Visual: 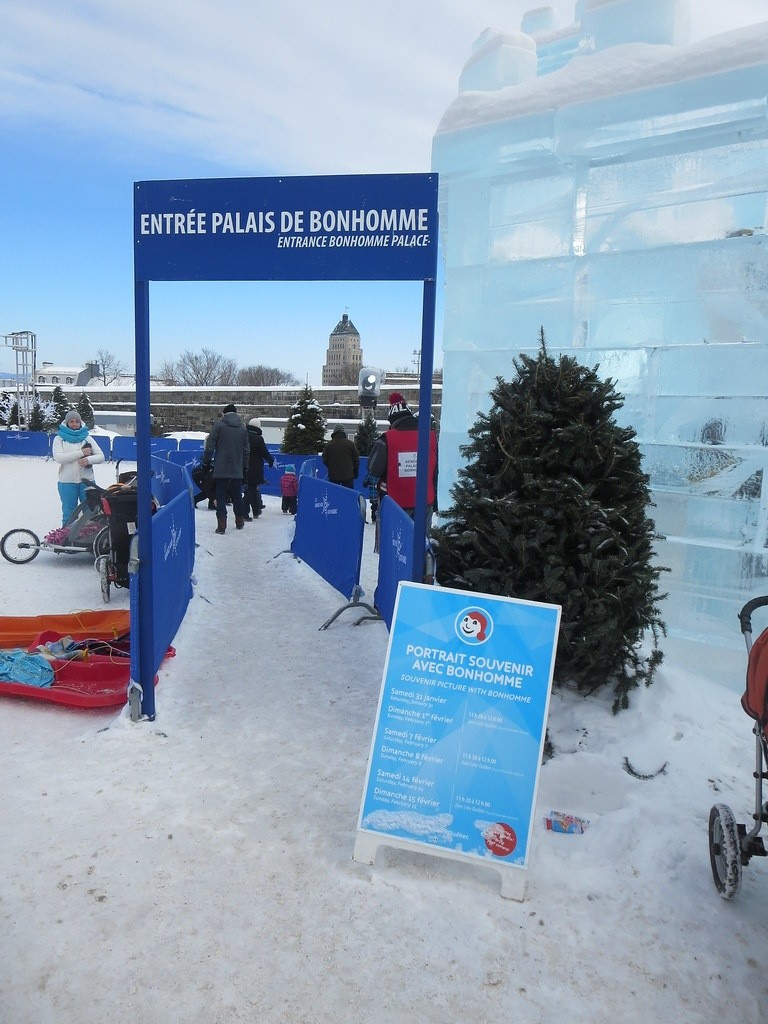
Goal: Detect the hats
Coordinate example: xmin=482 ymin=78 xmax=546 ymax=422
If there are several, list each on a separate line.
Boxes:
xmin=222 ymin=403 xmax=237 ymax=413
xmin=333 ymin=424 xmax=344 ymax=433
xmin=65 ymin=411 xmax=81 ymax=427
xmin=284 ymin=464 xmax=296 ymax=475
xmin=387 ymin=393 xmax=414 ymax=424
xmin=249 ymin=418 xmax=262 ymax=427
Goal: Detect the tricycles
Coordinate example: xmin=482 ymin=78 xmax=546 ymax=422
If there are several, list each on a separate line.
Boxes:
xmin=0 ymin=455 xmax=138 ymax=603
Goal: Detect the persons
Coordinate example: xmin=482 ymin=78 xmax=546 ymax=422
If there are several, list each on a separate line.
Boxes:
xmin=323 ymin=423 xmax=360 ymax=490
xmin=192 ymin=404 xmax=273 ymax=534
xmin=368 ymin=394 xmax=438 ymax=586
xmin=281 ymin=465 xmax=298 ymax=515
xmin=53 ymin=411 xmax=105 ymax=554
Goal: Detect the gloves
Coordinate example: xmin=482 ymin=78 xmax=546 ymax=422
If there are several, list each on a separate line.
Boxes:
xmin=268 ymin=461 xmax=275 ymax=468
xmin=353 ymin=468 xmax=359 ymax=479
xmin=243 ymin=470 xmax=249 ymax=480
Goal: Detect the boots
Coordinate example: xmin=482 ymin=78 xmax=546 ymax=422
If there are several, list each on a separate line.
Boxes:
xmin=215 ymin=518 xmax=226 ymax=533
xmin=236 ymin=515 xmax=244 ymax=529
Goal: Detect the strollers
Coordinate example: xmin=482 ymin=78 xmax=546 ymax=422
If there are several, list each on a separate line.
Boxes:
xmin=709 ymin=595 xmax=768 ymax=899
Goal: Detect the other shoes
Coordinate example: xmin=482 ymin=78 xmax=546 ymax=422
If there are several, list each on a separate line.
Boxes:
xmin=195 ymin=502 xmax=198 ymax=508
xmin=283 ymin=509 xmax=287 ymax=513
xmin=261 ymin=504 xmax=266 ymax=510
xmin=253 ymin=509 xmax=262 ymax=518
xmin=208 ymin=502 xmax=217 ymax=509
xmin=243 ymin=517 xmax=252 ymax=522
